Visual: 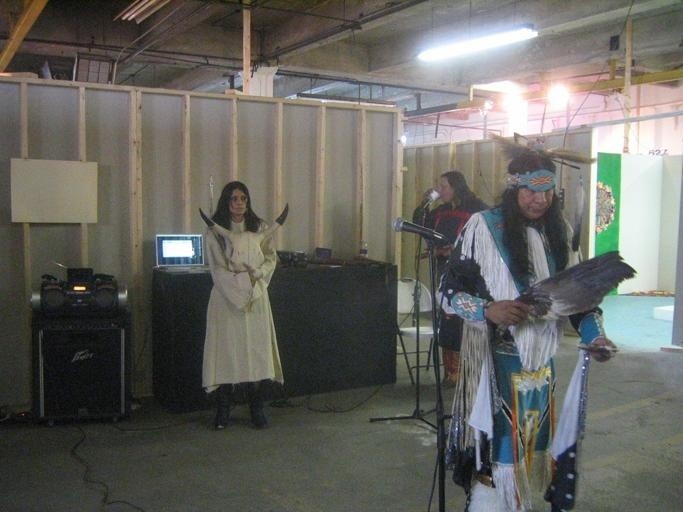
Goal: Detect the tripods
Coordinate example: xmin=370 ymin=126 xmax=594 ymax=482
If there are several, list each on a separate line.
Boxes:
xmin=368 ymin=242 xmax=440 ymax=431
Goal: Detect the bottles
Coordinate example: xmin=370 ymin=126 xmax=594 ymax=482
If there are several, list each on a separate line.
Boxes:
xmin=358 ymin=239 xmax=367 ymax=263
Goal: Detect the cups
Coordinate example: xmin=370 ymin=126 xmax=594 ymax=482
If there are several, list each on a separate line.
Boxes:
xmin=429 ymin=191 xmax=439 ymax=201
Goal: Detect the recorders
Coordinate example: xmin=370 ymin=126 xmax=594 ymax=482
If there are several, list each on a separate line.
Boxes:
xmin=31 ymin=267 xmax=128 ymax=318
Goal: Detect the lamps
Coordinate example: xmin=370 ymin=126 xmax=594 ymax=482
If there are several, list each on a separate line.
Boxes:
xmin=415 ymin=1 xmax=540 ymax=67
xmin=113 ymin=0 xmax=171 ymax=25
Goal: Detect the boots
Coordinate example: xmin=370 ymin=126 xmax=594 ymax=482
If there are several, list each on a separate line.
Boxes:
xmin=249 ymin=384 xmax=268 ymax=430
xmin=212 ymin=380 xmax=231 ymax=430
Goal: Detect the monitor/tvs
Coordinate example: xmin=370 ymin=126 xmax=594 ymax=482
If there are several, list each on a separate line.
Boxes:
xmin=155 ymin=232 xmax=204 ymax=273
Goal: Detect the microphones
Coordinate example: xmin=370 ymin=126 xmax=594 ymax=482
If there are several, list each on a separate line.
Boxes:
xmin=391 ymin=216 xmax=445 ymax=242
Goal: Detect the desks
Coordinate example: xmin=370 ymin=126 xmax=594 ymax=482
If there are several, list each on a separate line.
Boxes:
xmin=145 ymin=250 xmax=398 ymax=417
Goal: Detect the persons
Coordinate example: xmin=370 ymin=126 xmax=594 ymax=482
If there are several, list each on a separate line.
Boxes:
xmin=434 ymin=147 xmax=622 ymax=511
xmin=411 ymin=171 xmax=495 ymax=389
xmin=203 ymin=181 xmax=282 ymax=430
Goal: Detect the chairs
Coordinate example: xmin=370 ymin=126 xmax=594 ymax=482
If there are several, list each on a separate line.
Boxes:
xmin=393 ymin=274 xmax=445 ymax=392
